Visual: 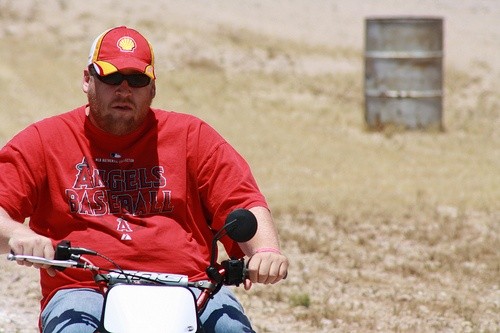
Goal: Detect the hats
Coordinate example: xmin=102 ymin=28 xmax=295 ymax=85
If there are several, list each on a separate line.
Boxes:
xmin=87 ymin=25 xmax=157 ymax=80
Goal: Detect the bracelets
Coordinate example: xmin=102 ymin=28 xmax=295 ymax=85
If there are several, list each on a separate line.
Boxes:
xmin=251 ymin=248 xmax=282 ymax=255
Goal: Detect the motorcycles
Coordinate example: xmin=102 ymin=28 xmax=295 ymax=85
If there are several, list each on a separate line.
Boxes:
xmin=7 ymin=208 xmax=287 ymax=333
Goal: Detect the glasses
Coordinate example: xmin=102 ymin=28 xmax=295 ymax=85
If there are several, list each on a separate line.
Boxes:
xmin=95 ymin=72 xmax=151 ymax=88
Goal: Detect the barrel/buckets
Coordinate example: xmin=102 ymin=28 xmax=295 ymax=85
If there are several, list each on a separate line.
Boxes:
xmin=365 ymin=18 xmax=444 ymax=130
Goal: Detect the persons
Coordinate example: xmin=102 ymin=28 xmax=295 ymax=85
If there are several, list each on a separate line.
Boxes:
xmin=0 ymin=26 xmax=288 ymax=333
